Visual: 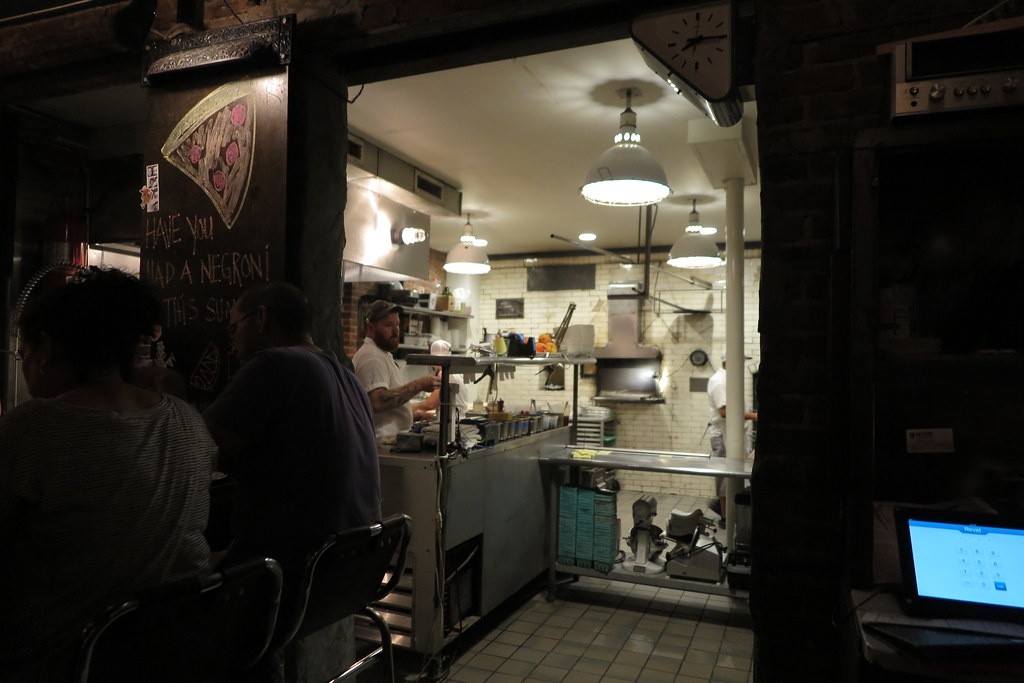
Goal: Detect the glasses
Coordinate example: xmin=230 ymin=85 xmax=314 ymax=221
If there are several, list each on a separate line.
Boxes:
xmin=226 ymin=310 xmax=257 ymax=335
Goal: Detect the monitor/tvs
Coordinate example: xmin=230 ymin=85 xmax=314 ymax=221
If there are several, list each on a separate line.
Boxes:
xmin=892 ymin=505 xmax=1024 ymax=625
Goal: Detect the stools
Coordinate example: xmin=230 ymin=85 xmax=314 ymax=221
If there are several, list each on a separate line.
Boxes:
xmin=264 ymin=512 xmax=413 ymax=683
xmin=70 ymin=556 xmax=284 ymax=683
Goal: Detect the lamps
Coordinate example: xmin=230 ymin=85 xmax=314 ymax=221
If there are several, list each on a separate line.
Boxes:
xmin=442 ymin=212 xmax=491 ymax=275
xmin=665 ymin=199 xmax=725 ymax=270
xmin=577 ymin=87 xmax=673 ymax=207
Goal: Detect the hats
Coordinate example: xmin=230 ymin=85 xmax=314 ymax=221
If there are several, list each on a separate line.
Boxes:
xmin=721 ymin=349 xmax=752 ymax=362
xmin=363 ymin=300 xmax=403 ymax=320
xmin=431 ymin=339 xmax=452 ymax=356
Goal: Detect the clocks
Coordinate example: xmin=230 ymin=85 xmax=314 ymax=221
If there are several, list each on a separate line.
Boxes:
xmin=628 ymin=0 xmax=744 ymax=128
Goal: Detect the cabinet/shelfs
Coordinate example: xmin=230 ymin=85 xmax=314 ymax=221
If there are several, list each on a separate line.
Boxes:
xmin=356 ymin=302 xmax=475 ymax=360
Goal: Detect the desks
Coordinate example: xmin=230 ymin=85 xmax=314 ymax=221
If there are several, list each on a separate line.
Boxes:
xmin=538 ymin=447 xmax=753 ymax=603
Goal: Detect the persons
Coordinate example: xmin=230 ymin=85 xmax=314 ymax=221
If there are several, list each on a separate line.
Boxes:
xmin=411 ymin=341 xmax=469 ymax=421
xmin=0 ymin=267 xmax=381 ymax=683
xmin=706 ymin=351 xmax=758 ymax=529
xmin=354 ymin=300 xmax=442 ymax=439
